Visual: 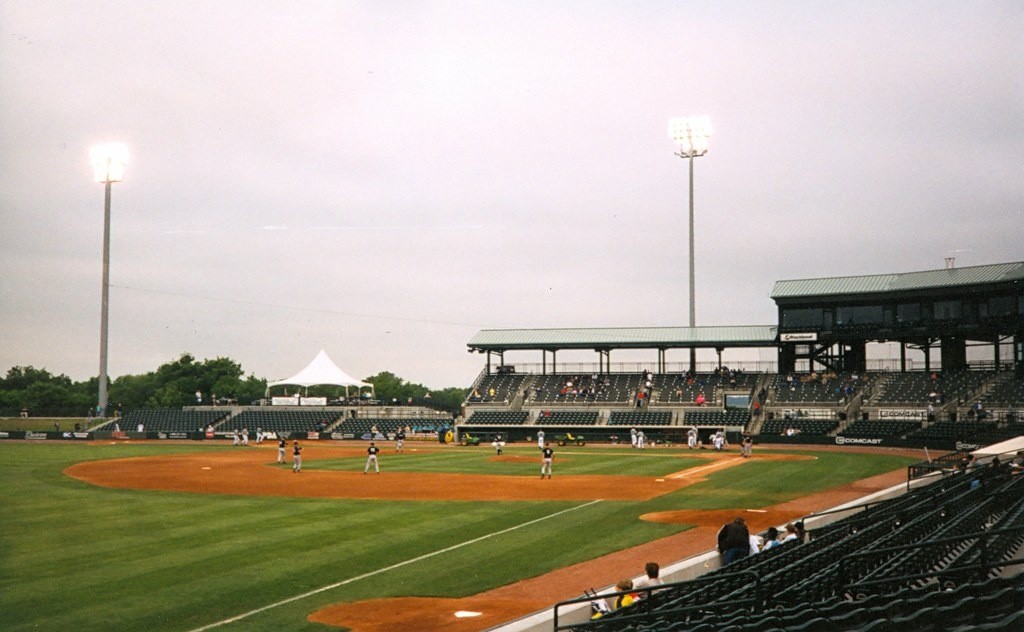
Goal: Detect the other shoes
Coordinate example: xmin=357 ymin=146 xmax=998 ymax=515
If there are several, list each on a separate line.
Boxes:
xmin=540 ymin=474 xmax=544 ymax=479
xmin=256 ymin=442 xmax=263 ymax=445
xmin=277 ymin=462 xmax=286 ymax=464
xmin=396 ymin=450 xmax=403 ymax=453
xmin=377 ymin=472 xmax=379 ymax=475
xmin=637 ymin=448 xmax=646 ymax=450
xmin=689 ymin=447 xmax=693 ymax=449
xmin=548 ymin=475 xmax=552 ymax=479
xmin=539 ymin=447 xmax=542 ymax=451
xmin=363 ymin=471 xmax=366 ymax=474
xmin=293 ymin=470 xmax=303 ymax=473
xmin=632 ymin=445 xmax=635 ymax=447
xmin=233 ymin=442 xmax=247 ymax=446
xmin=497 ymin=449 xmax=504 ymax=455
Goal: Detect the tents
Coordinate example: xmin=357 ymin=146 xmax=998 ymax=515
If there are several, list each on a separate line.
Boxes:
xmin=266 ymin=350 xmax=374 ymax=406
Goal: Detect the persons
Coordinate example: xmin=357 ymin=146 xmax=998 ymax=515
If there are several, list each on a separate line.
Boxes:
xmin=365 ymin=442 xmax=379 ymax=473
xmin=371 ymin=425 xmax=410 ymax=453
xmin=256 ymin=424 xmax=263 ymax=444
xmin=614 ymin=563 xmax=664 ymax=611
xmin=523 ymin=386 xmax=545 ymax=404
xmin=293 ymin=442 xmax=302 ymax=472
xmin=493 ymin=438 xmax=502 ymax=454
xmin=233 ymin=425 xmax=249 ymax=446
xmin=212 ymin=393 xmax=232 ymax=406
xmin=561 ymin=372 xmax=610 ymax=398
xmin=537 ymin=429 xmax=553 ymax=479
xmin=278 ymin=437 xmax=287 ymax=463
xmin=754 ymin=370 xmax=867 ymax=437
xmin=928 ymin=402 xmax=934 ymax=413
xmin=362 ymin=390 xmax=370 ymax=401
xmin=630 ymin=427 xmax=644 ymax=448
xmin=974 ymin=400 xmax=983 ymax=411
xmin=638 ymin=365 xmax=740 ymax=408
xmin=20 ymin=403 xmax=144 ymax=432
xmin=688 ymin=424 xmax=753 ymax=459
xmin=716 ymin=518 xmax=804 ymax=564
xmin=196 ymin=390 xmax=202 ymax=403
xmin=207 ymin=425 xmax=214 ymax=432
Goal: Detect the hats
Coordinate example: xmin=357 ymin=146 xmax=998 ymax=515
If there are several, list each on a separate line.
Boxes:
xmin=785 ymin=523 xmax=793 ymax=531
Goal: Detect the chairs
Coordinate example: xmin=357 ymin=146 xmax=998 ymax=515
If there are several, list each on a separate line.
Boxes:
xmin=574 ymin=469 xmax=1024 ymax=632
xmin=465 ymin=368 xmax=1024 ymax=448
xmin=95 ymin=406 xmax=455 ymax=433
